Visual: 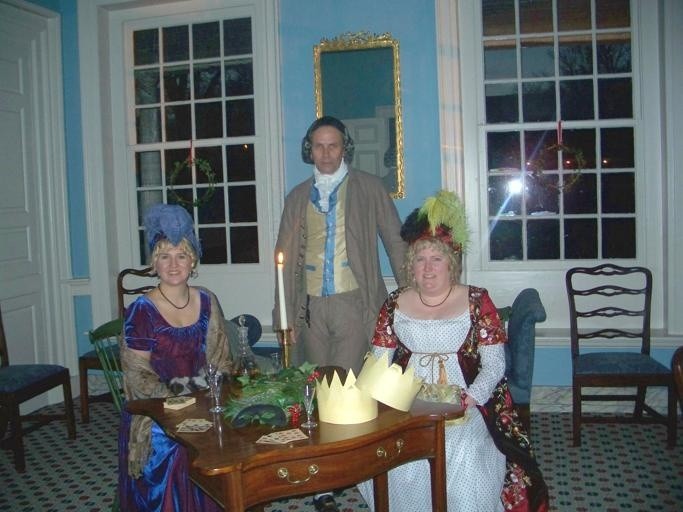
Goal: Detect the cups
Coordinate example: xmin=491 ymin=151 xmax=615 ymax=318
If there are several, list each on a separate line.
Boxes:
xmin=271 ymin=352 xmax=282 ymax=368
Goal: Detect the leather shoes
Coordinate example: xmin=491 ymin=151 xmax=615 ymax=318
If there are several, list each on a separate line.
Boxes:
xmin=312 ymin=493 xmax=340 ymax=512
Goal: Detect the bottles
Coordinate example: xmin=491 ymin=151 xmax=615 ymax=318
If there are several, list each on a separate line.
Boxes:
xmin=226 ymin=316 xmax=261 ymax=404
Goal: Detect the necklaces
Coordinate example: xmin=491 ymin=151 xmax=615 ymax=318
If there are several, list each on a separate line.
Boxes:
xmin=157 ymin=283 xmax=191 ymax=309
xmin=416 ymin=288 xmax=452 ymax=306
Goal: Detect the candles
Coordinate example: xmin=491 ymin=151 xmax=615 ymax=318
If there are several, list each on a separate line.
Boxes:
xmin=276 ymin=250 xmax=288 ymax=331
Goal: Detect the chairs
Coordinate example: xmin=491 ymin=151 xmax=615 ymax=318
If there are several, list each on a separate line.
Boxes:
xmin=565 ymin=261 xmax=679 ymax=450
xmin=87 ymin=316 xmax=129 ymax=511
xmin=211 ymin=292 xmax=283 ymax=379
xmin=497 ymin=287 xmax=547 ymax=445
xmin=78 ymin=263 xmax=160 ymax=426
xmin=0 ymin=308 xmax=76 ymax=474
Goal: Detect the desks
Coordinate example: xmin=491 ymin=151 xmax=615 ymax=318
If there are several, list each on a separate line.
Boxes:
xmin=123 ymin=364 xmax=465 ymax=512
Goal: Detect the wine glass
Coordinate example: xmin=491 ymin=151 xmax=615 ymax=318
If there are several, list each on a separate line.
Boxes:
xmin=201 ymin=361 xmax=225 ymax=413
xmin=301 ymin=385 xmax=317 ymax=429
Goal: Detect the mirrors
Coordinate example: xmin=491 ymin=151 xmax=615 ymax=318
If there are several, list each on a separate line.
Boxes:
xmin=310 ymin=27 xmax=407 ymax=202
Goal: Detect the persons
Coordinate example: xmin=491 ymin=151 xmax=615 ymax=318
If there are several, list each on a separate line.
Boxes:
xmin=273 ymin=115 xmax=409 ymax=511
xmin=113 ymin=203 xmax=272 ymax=512
xmin=355 ymin=188 xmax=549 ymax=512
